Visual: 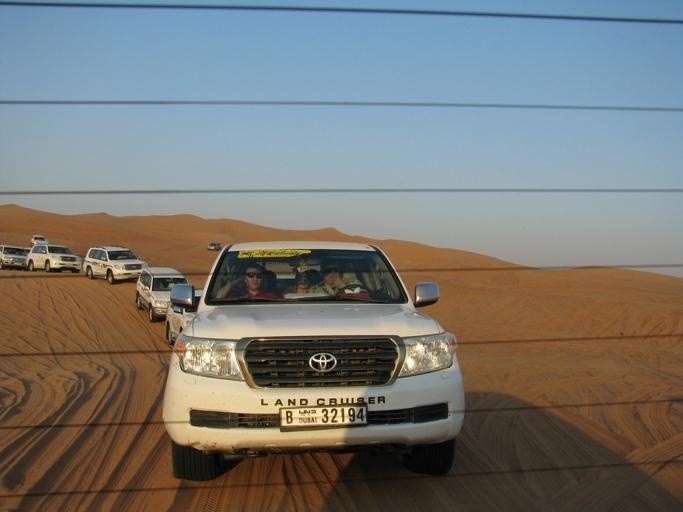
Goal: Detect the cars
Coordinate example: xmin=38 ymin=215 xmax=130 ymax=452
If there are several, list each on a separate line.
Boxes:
xmin=29 ymin=235 xmax=47 ymax=245
xmin=207 ymin=242 xmax=220 ymax=251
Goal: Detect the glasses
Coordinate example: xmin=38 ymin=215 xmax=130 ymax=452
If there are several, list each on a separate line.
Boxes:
xmin=246 ymin=272 xmax=263 ymax=278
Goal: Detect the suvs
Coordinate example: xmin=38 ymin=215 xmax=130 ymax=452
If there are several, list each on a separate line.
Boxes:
xmin=0 ymin=245 xmax=30 ymax=269
xmin=135 ymin=266 xmax=188 ymax=323
xmin=162 ymin=289 xmax=204 ymax=345
xmin=24 ymin=244 xmax=81 ymax=273
xmin=160 ymin=241 xmax=466 ymax=480
xmin=82 ymin=246 xmax=147 ymax=285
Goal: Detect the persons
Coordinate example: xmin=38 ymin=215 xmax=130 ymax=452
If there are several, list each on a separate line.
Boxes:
xmin=215 ymin=263 xmax=277 ymax=301
xmin=278 ymin=262 xmax=321 ymax=300
xmin=309 ymin=257 xmax=365 ymax=298
